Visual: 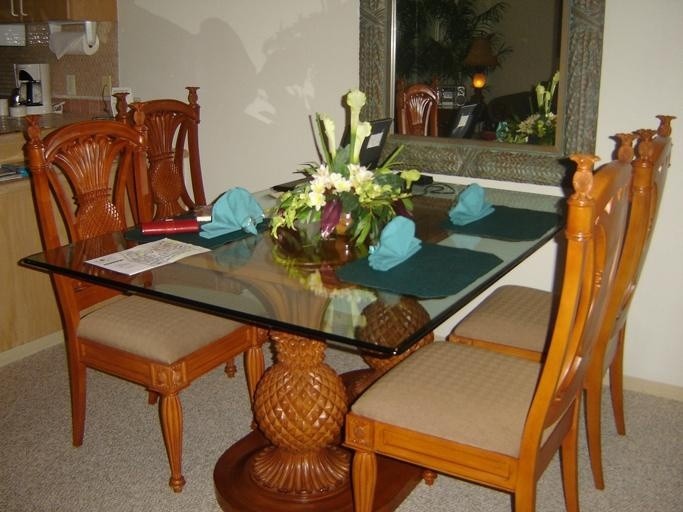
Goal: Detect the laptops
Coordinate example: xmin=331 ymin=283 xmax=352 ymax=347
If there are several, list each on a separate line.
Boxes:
xmin=451 ymin=104 xmax=477 ymax=136
xmin=273 ymin=118 xmax=392 ymax=192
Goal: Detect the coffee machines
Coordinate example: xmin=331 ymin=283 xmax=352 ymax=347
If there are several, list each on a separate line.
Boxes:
xmin=14 ymin=63 xmax=52 ymax=115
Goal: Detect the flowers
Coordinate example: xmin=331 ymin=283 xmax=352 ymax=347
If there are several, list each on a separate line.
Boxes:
xmin=516 ymin=70 xmax=559 ymax=139
xmin=271 ymin=90 xmax=419 ymax=253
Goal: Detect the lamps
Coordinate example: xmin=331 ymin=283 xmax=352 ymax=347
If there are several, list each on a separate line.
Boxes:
xmin=460 ymin=37 xmax=499 ymax=123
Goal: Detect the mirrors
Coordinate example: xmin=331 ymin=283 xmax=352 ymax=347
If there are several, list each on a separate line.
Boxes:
xmin=357 ymin=0 xmax=606 ymax=188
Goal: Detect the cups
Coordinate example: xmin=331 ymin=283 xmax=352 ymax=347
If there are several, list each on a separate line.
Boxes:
xmin=1 ymin=99 xmax=9 ymax=116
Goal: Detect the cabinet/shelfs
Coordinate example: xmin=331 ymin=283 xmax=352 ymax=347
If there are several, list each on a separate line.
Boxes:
xmin=0 ymin=142 xmax=190 ymax=368
xmin=0 ymin=0 xmax=116 ymax=26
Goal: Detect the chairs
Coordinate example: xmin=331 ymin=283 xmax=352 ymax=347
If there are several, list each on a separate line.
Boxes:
xmin=343 ymin=131 xmax=639 ymax=512
xmin=394 ymin=75 xmax=440 ymax=139
xmin=109 ymin=86 xmax=236 ymax=377
xmin=23 ymin=101 xmax=269 ymax=492
xmin=445 ymin=114 xmax=677 ymax=491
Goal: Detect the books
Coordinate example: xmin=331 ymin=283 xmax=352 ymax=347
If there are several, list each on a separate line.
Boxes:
xmin=195 ymin=203 xmax=211 ymax=222
xmin=0 ymin=163 xmax=29 ymax=182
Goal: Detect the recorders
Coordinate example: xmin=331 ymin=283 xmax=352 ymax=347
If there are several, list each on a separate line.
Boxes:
xmin=436 ymin=85 xmax=465 ymax=107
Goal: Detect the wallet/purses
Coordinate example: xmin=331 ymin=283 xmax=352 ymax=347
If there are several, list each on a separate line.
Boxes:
xmin=142 ymin=220 xmax=200 ymax=236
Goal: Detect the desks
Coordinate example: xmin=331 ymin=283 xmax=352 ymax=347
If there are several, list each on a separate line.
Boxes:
xmin=17 ymin=168 xmax=569 ymax=511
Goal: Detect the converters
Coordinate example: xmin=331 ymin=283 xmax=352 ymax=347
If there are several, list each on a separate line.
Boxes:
xmin=397 ymin=172 xmax=433 ymax=185
